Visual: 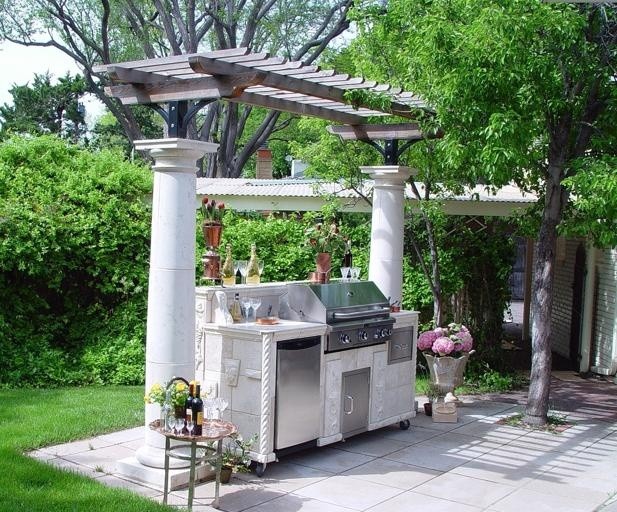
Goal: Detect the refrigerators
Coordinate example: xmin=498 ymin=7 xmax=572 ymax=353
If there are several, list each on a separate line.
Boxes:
xmin=274 ymin=335 xmax=322 ymax=450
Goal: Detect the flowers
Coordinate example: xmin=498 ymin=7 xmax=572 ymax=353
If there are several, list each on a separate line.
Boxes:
xmin=201 ymin=197 xmax=224 ymax=225
xmin=145 ymin=380 xmax=189 ymax=416
xmin=417 ymin=322 xmax=474 ymax=358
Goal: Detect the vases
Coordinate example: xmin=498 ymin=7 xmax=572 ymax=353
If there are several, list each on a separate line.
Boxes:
xmin=202 ymin=223 xmax=221 ymax=247
xmin=158 ymin=405 xmax=173 ymax=427
xmin=425 ymin=351 xmax=472 ymax=402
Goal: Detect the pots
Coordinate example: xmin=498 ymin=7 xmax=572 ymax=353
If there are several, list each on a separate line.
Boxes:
xmin=257 ymin=304 xmax=280 ymax=325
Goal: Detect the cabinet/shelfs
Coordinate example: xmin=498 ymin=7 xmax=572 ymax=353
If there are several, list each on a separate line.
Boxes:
xmin=369 ymin=352 xmax=417 ymax=432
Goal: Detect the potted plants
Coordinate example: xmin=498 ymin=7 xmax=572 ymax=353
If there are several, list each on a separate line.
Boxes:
xmin=301 ymin=224 xmax=347 ymax=282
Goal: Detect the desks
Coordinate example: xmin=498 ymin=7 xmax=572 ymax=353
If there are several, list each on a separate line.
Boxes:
xmin=150 ymin=419 xmax=238 ymax=512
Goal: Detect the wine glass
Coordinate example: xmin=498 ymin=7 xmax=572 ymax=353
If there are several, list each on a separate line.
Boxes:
xmin=238 ymin=261 xmax=248 ymax=287
xmin=203 ymin=396 xmax=229 ymax=432
xmin=242 ymin=296 xmax=261 ymax=324
xmin=167 ymin=417 xmax=194 ymax=437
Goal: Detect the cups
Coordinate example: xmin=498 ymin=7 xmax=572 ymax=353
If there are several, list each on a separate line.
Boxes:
xmin=339 ymin=267 xmax=361 ymax=282
xmin=257 ymin=260 xmax=265 ymax=276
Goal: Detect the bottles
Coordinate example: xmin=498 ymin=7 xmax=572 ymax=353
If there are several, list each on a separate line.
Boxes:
xmin=184 ymin=384 xmax=203 ymax=437
xmin=230 ymin=293 xmax=242 ymax=321
xmin=344 ymin=239 xmax=353 ymax=267
xmin=222 ymin=245 xmax=235 ymax=286
xmin=246 ymin=244 xmax=260 ymax=286
